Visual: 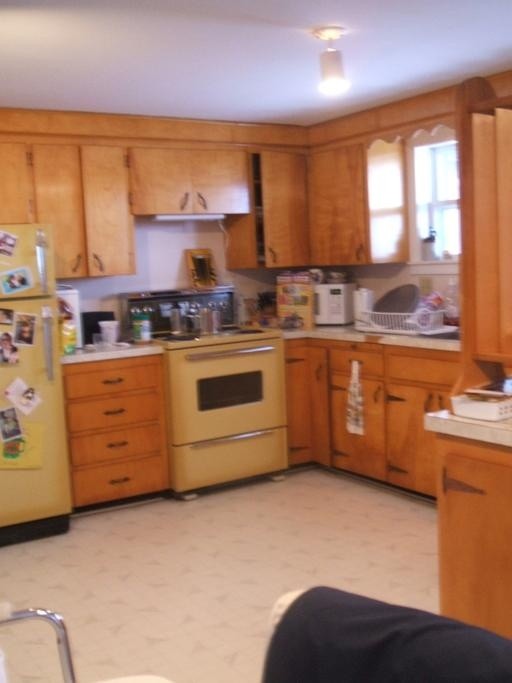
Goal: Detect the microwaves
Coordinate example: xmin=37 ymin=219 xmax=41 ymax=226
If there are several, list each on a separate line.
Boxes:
xmin=314 ymin=280 xmax=360 ymax=325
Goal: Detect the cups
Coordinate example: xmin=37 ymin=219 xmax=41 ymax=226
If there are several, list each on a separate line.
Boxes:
xmin=99 ymin=321 xmax=119 ymax=344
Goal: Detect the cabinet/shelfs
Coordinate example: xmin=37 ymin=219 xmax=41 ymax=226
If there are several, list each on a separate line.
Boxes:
xmin=386 ymin=347 xmax=461 ymax=500
xmin=284 ymin=337 xmax=387 ymax=482
xmin=63 ymin=353 xmax=171 ymax=513
xmin=0 ymin=102 xmax=408 ymax=278
xmin=434 ymin=433 xmax=512 ymax=646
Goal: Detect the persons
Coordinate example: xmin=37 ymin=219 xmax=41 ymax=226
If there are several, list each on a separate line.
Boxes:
xmin=16 ymin=320 xmax=32 ymax=344
xmin=7 ymin=274 xmax=23 ymax=288
xmin=0 ymin=332 xmax=17 ymax=362
xmin=0 ymin=411 xmax=19 ymax=436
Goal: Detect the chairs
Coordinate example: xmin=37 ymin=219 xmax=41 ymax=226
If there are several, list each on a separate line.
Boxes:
xmin=0 ymin=609 xmax=175 ymax=683
xmin=263 ymin=587 xmax=512 ymax=683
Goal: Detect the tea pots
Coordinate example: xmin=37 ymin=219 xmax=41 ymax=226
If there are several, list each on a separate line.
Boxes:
xmin=185 ymin=301 xmax=201 ymax=333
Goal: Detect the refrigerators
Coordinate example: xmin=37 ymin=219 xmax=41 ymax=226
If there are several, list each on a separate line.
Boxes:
xmin=1 ymin=223 xmax=74 ymax=549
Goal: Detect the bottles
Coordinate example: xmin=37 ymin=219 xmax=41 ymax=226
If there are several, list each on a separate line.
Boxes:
xmin=131 ymin=312 xmax=153 ymax=345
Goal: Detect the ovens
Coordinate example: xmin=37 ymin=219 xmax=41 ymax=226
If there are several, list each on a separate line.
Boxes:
xmin=166 ymin=336 xmax=289 ymax=493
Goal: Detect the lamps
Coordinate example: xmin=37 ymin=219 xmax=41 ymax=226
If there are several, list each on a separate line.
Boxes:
xmin=315 ymin=27 xmax=350 ymax=95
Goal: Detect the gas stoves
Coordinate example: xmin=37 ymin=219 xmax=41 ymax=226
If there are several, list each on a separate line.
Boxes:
xmin=149 ymin=325 xmax=266 ymax=351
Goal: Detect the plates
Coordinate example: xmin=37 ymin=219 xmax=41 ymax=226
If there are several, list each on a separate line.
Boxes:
xmin=370 ymin=282 xmax=421 ymax=331
